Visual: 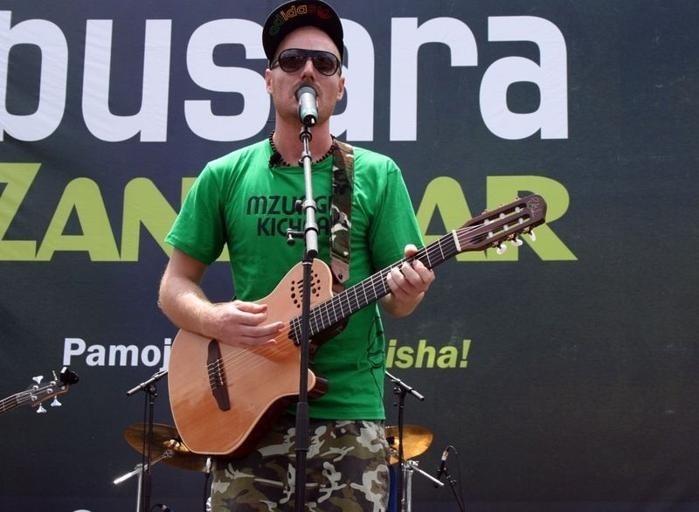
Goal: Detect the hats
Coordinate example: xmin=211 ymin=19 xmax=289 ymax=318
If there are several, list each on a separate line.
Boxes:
xmin=262 ymin=0 xmax=343 ymax=65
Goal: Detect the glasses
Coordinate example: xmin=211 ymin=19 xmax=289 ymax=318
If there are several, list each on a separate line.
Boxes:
xmin=271 ymin=48 xmax=341 ymax=77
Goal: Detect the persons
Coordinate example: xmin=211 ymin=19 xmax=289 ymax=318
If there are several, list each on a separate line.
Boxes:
xmin=157 ymin=0 xmax=435 ymax=512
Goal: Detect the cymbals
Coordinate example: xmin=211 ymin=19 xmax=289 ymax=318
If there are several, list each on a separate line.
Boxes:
xmin=385 ymin=425 xmax=433 ymax=467
xmin=125 ymin=422 xmax=217 ymax=474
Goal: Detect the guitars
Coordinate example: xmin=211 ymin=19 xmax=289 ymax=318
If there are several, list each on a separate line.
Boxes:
xmin=0 ymin=365 xmax=79 ymax=415
xmin=168 ymin=195 xmax=547 ymax=454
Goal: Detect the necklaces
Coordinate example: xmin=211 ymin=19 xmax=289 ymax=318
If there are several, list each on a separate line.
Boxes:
xmin=268 ymin=129 xmax=336 ymax=167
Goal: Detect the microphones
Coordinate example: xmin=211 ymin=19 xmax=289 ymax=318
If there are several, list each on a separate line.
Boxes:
xmin=432 ymin=447 xmax=450 ymax=488
xmin=295 ymin=85 xmax=319 ymax=127
